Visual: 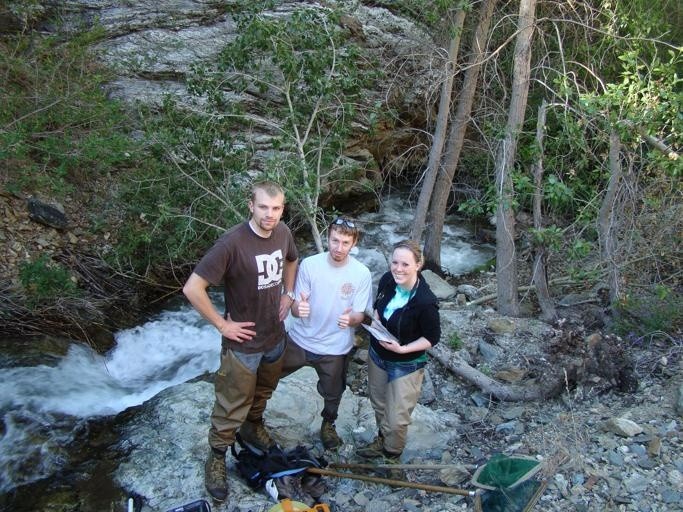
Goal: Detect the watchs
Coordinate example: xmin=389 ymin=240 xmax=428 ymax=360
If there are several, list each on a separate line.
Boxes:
xmin=283 ymin=288 xmax=297 ymax=301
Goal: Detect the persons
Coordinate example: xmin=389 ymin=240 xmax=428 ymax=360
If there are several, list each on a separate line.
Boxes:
xmin=341 ymin=239 xmax=442 ymax=491
xmin=183 ymin=180 xmax=299 ymax=502
xmin=280 ymin=216 xmax=374 ymax=451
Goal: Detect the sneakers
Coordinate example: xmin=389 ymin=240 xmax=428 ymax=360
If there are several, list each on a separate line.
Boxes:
xmin=238 ymin=419 xmax=275 ymax=452
xmin=383 ymin=455 xmax=404 ymax=491
xmin=321 ymin=419 xmax=339 ymax=449
xmin=355 ymin=436 xmax=384 ymax=457
xmin=274 ymin=473 xmax=326 ymax=508
xmin=205 ymin=449 xmax=226 ymax=502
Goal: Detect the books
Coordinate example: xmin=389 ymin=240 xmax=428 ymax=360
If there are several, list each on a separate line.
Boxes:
xmin=359 ymin=311 xmax=401 ymax=344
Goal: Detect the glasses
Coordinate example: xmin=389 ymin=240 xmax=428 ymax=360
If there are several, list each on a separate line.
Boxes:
xmin=331 ymin=218 xmax=357 ymax=228
